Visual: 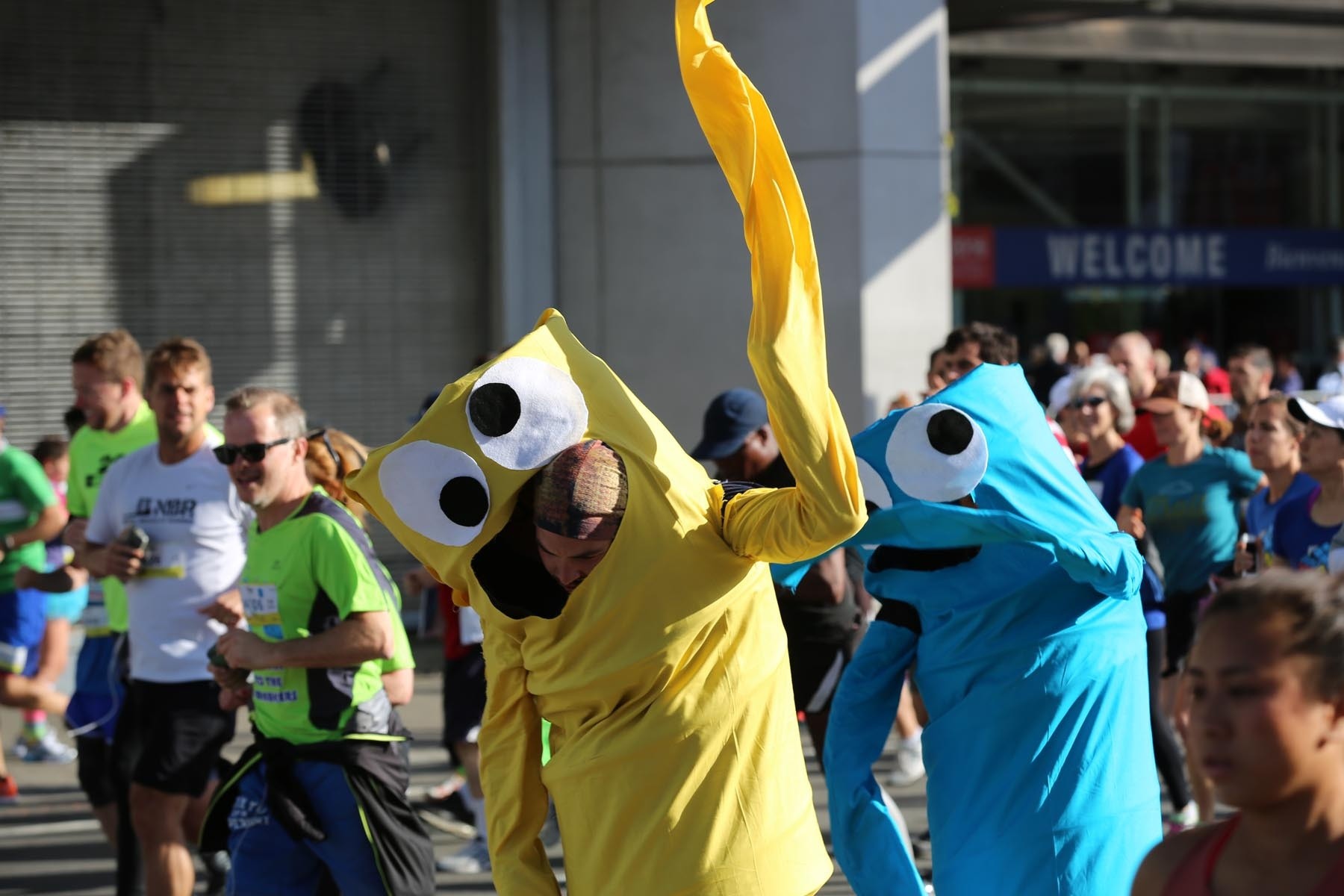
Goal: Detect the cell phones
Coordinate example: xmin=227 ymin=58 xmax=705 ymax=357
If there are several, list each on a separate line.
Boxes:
xmin=1242 ymin=532 xmax=1258 ymax=577
xmin=117 ymin=527 xmax=150 ymax=585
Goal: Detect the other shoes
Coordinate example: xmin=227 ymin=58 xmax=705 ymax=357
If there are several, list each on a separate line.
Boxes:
xmin=886 ymin=727 xmax=925 ymax=789
xmin=538 ymin=819 xmax=565 ymax=860
xmin=1159 ymin=800 xmax=1201 ymax=839
xmin=15 ymin=732 xmax=78 ymax=762
xmin=405 ymin=783 xmax=478 ymax=840
xmin=434 ymin=835 xmax=491 ymax=874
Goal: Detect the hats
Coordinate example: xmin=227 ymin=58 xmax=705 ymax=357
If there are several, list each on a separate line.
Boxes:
xmin=691 ymin=388 xmax=768 ymax=460
xmin=1046 ymin=375 xmax=1072 ymax=419
xmin=1135 ymin=371 xmax=1210 ymax=420
xmin=1286 ymin=392 xmax=1344 ymax=428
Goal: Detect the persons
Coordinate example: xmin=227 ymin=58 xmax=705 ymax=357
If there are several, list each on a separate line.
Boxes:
xmin=0 ymin=318 xmax=1344 ymax=895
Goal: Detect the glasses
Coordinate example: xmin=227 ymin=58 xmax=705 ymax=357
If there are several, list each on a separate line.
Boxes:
xmin=1075 ymin=396 xmax=1109 ymax=409
xmin=212 ymin=437 xmax=296 ymax=465
xmin=305 ymin=427 xmax=343 ymax=480
